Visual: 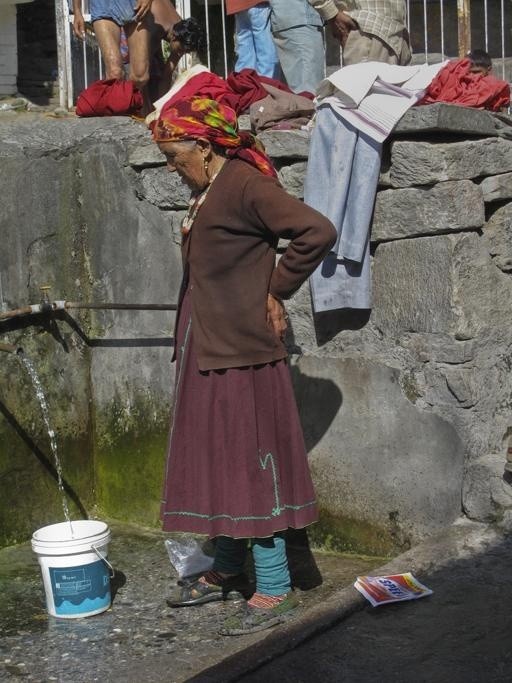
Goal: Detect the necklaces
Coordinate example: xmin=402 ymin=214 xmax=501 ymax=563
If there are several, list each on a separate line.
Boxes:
xmin=180 ymin=173 xmax=216 ymax=234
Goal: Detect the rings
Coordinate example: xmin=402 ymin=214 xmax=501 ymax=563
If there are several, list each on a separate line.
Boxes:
xmin=284 ymin=316 xmax=289 ymax=322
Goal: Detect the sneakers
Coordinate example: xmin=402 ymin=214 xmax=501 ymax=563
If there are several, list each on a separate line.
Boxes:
xmin=166 ymin=574 xmax=249 ymax=606
xmin=217 ymin=593 xmax=301 ymax=636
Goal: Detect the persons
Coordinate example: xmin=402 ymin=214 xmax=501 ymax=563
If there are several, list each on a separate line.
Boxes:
xmin=153 ymin=96 xmax=338 ymax=636
xmin=464 ymin=50 xmax=494 ymax=79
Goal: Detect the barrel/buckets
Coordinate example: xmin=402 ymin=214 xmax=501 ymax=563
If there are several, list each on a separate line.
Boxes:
xmin=31 ymin=520 xmax=116 ymax=620
